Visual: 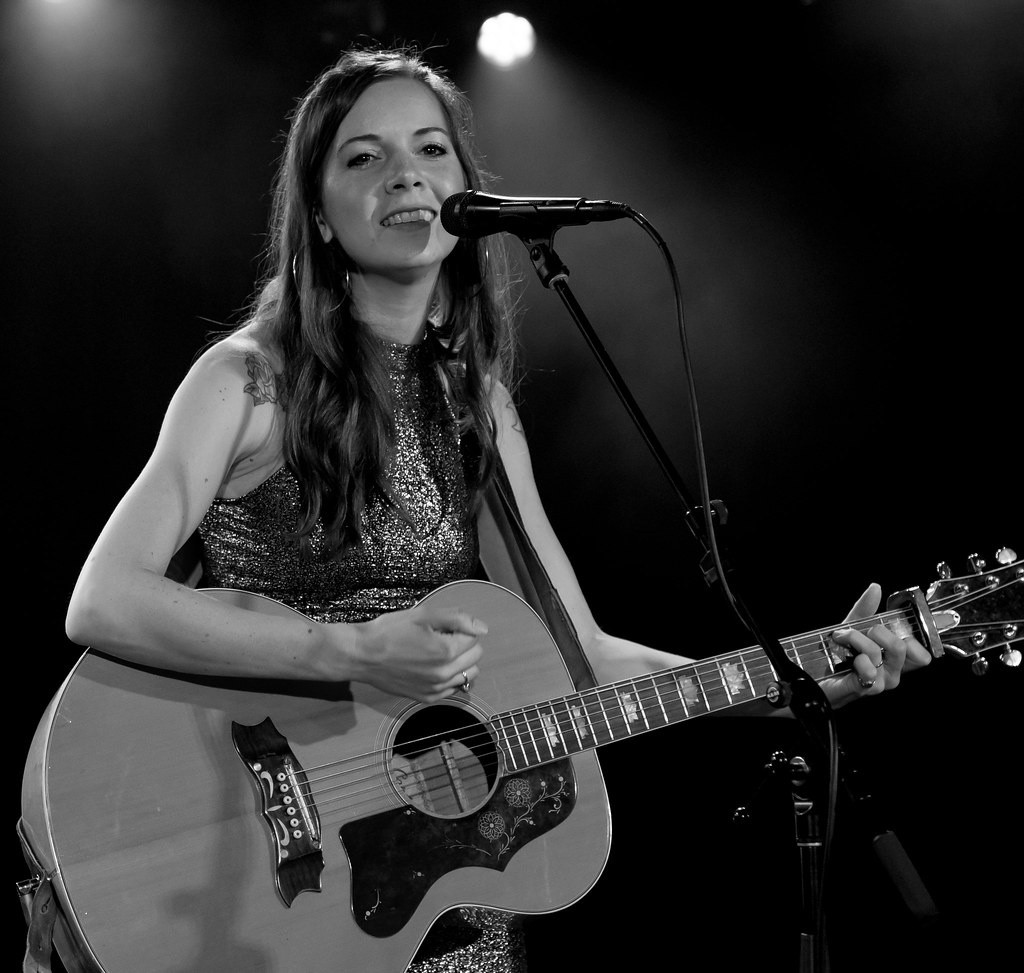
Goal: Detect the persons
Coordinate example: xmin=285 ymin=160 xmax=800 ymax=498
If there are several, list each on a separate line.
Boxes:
xmin=66 ymin=52 xmax=935 ymax=973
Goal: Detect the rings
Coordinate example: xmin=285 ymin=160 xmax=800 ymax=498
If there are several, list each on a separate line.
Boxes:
xmin=461 ymin=672 xmax=471 ymax=694
xmin=858 ymin=676 xmax=876 ymax=688
xmin=874 ymin=661 xmax=883 ymax=669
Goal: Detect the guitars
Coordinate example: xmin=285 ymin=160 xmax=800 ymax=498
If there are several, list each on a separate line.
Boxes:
xmin=17 ymin=541 xmax=1024 ymax=971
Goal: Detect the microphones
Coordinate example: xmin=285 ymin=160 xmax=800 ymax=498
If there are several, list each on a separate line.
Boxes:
xmin=440 ymin=190 xmax=630 ymax=239
